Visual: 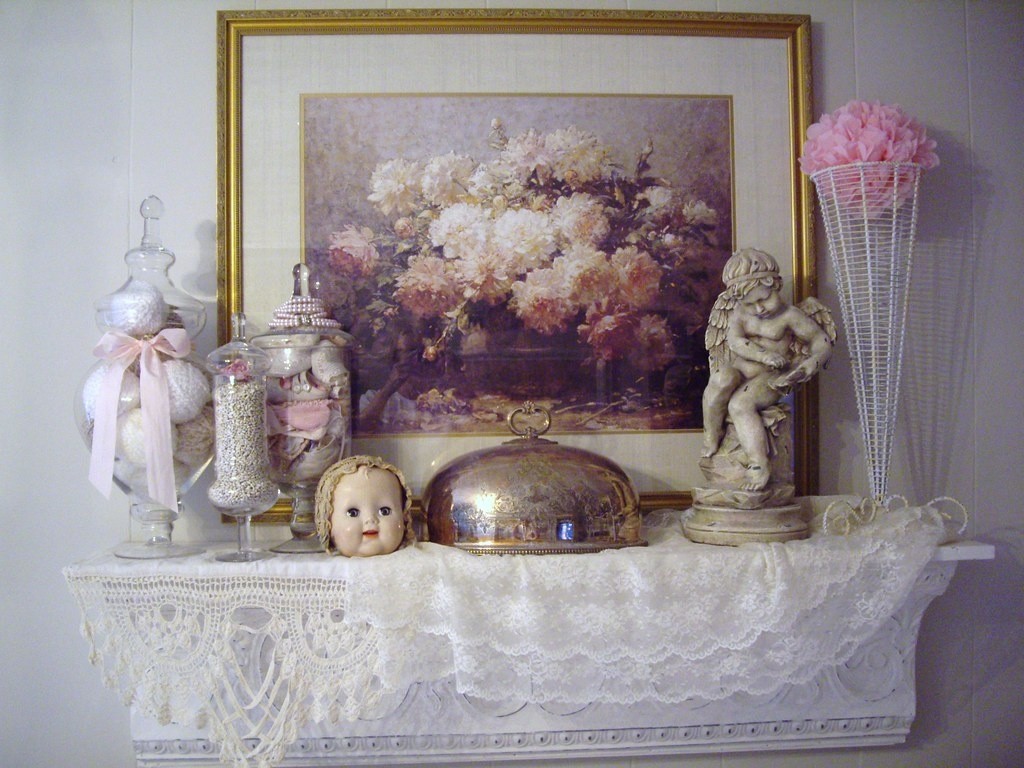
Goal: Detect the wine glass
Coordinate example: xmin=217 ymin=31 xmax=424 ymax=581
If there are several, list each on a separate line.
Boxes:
xmin=80 ymin=197 xmax=218 ymax=562
xmin=202 ymin=316 xmax=278 ymax=563
xmin=253 ymin=261 xmax=352 ymax=558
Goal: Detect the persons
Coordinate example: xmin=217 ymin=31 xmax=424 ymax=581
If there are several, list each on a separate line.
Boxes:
xmin=314 ymin=455 xmax=416 ymax=558
xmin=696 ymin=244 xmax=837 ymax=494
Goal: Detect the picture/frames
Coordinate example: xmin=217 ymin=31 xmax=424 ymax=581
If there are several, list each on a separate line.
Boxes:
xmin=215 ymin=6 xmax=817 ymax=524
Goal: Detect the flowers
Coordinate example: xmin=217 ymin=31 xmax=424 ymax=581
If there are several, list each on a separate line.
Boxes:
xmin=795 ymin=96 xmax=939 ymax=219
